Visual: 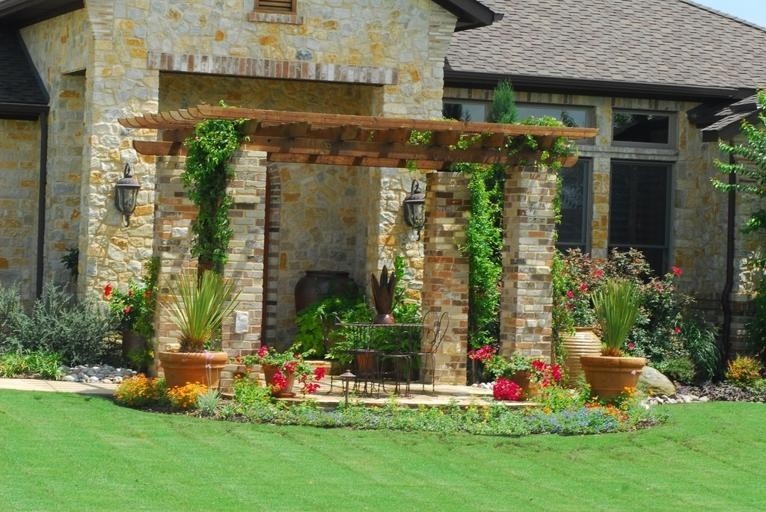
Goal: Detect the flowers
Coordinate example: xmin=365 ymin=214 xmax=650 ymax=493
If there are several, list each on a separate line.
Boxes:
xmin=467 ymin=344 xmax=563 ymax=401
xmin=560 ymin=247 xmax=606 ymax=325
xmin=104 ymin=285 xmax=152 ymax=337
xmin=244 ymin=343 xmax=329 ymax=395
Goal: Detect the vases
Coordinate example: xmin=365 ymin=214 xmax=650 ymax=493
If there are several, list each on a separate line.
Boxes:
xmin=264 ymin=364 xmax=293 ymax=397
xmin=122 ymin=333 xmax=146 ymax=368
xmin=561 ymin=324 xmax=604 ymax=389
xmin=511 ymin=371 xmax=532 ymax=390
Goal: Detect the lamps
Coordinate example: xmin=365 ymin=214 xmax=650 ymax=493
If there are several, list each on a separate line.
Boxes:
xmin=403 ymin=180 xmax=427 ymax=240
xmin=116 ymin=164 xmax=140 ymax=225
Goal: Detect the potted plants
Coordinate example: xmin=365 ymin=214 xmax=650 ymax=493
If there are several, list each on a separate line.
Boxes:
xmin=580 ymin=279 xmax=647 ymax=404
xmin=150 ymin=270 xmax=242 ymax=387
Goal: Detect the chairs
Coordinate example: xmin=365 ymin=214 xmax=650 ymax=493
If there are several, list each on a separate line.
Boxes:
xmin=320 ymin=310 xmax=449 ymax=398
